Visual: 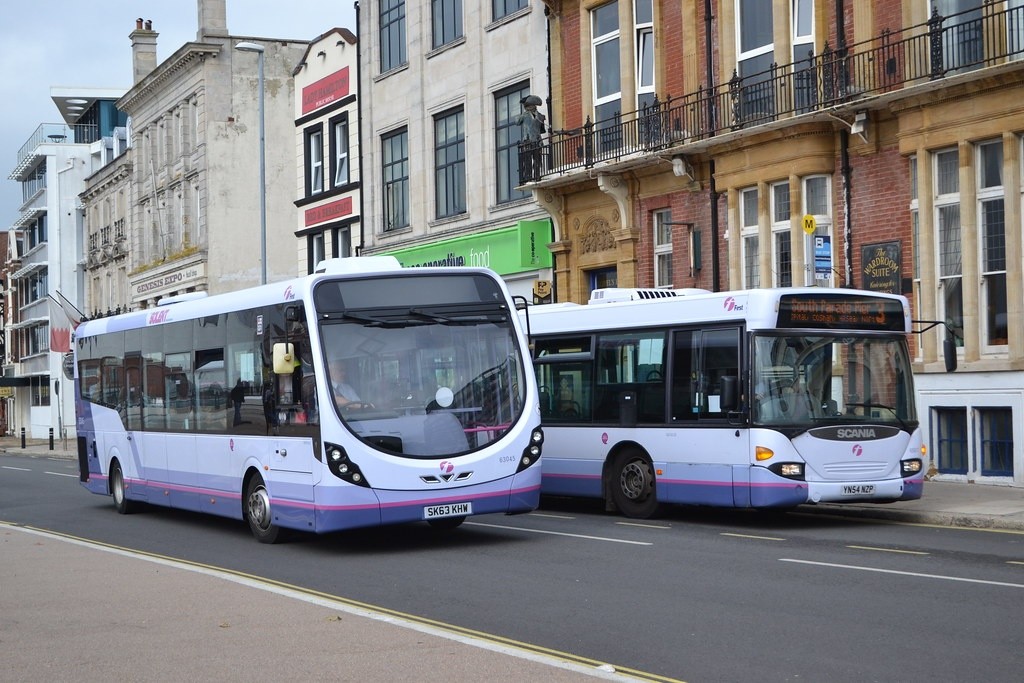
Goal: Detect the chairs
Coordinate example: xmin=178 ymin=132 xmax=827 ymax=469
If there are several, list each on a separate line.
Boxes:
xmin=537 ymin=386 xmax=581 ymax=421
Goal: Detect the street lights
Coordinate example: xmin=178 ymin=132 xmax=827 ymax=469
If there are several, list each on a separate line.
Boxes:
xmin=234 ymin=41 xmax=267 ymax=285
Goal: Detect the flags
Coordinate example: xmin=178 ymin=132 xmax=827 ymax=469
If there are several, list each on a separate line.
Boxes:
xmin=56 ymin=291 xmax=81 ymax=331
xmin=48 ymin=296 xmax=70 ymax=352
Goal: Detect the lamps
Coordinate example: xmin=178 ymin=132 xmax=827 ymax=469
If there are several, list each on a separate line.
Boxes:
xmin=671 ymin=154 xmax=696 ymax=182
xmin=850 ymin=110 xmax=869 ymax=134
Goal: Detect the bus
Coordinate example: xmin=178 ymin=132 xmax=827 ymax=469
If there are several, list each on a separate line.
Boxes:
xmin=516 ymin=287 xmax=961 ymax=522
xmin=73 ymin=256 xmax=545 ymax=546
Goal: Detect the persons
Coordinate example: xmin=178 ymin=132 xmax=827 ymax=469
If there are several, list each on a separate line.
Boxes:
xmin=89 ymin=367 xmax=126 ymax=413
xmin=80 ymin=304 xmax=120 ymax=322
xmin=741 ymin=369 xmax=764 ymax=402
xmin=516 ymin=95 xmax=546 ymax=181
xmin=231 ymin=378 xmax=245 ymax=427
xmin=314 ymin=360 xmax=375 ymax=422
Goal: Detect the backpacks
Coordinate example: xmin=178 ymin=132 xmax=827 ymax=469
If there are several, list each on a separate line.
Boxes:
xmin=231 ymin=389 xmax=237 ymax=400
